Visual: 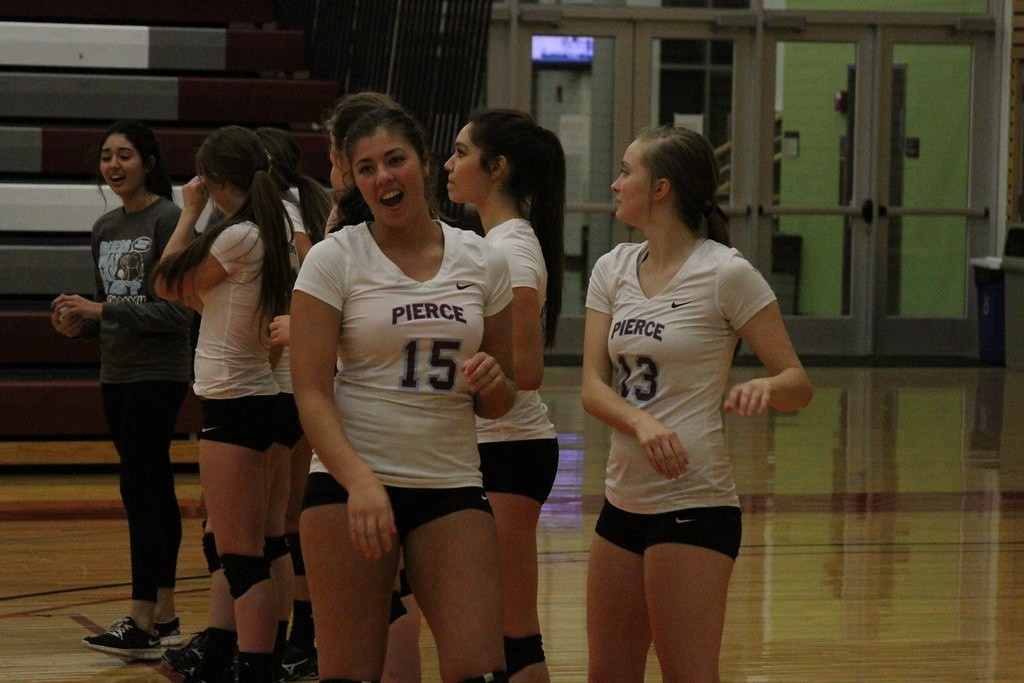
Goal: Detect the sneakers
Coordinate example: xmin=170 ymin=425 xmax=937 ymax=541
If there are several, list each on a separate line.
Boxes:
xmin=162 ymin=629 xmax=238 ymax=671
xmin=153 ymin=617 xmax=182 ymax=645
xmin=177 ymin=656 xmax=276 ymax=683
xmin=82 ymin=617 xmax=162 ymax=659
xmin=278 ymin=647 xmax=319 ymax=683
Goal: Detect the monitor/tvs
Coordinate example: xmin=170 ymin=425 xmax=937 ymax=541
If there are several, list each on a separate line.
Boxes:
xmin=530 ymin=34 xmax=593 ymax=70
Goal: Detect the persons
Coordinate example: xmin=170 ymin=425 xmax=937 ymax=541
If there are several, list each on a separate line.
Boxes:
xmin=582 ymin=128 xmax=815 ymax=683
xmin=48 ymin=92 xmax=565 ymax=683
